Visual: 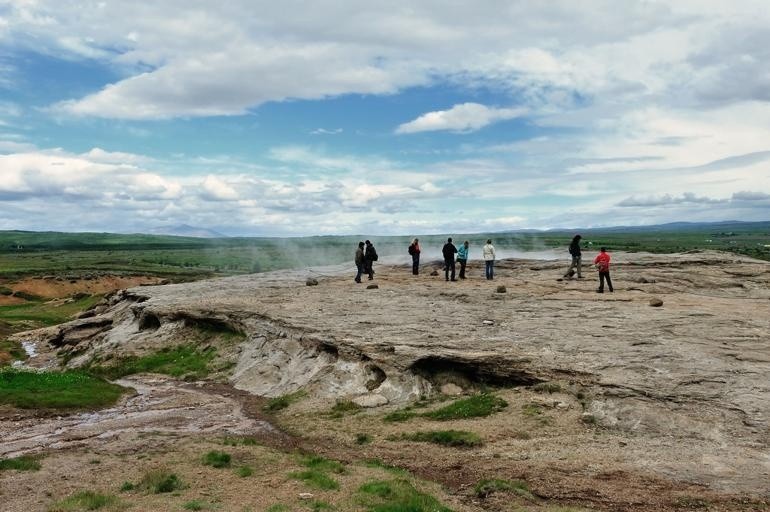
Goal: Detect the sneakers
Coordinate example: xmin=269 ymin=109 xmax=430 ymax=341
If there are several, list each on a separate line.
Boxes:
xmin=578 ymin=276 xmax=585 ymax=278
xmin=368 ymin=276 xmax=373 ymax=280
xmin=597 ymin=290 xmax=603 ymax=293
xmin=610 ymin=289 xmax=613 ymax=292
xmin=355 ymin=279 xmax=361 ymax=283
xmin=446 ymin=276 xmax=467 ymax=281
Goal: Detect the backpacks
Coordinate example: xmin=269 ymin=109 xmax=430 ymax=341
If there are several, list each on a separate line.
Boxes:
xmin=409 ymin=244 xmax=417 ymax=254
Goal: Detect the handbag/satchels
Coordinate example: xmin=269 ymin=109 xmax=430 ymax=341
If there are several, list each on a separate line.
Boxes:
xmin=372 ymin=254 xmax=377 ymax=261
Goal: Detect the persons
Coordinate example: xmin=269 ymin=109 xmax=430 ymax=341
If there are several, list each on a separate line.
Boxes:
xmin=594 ymin=247 xmax=613 ymax=293
xmin=482 ymin=238 xmax=496 ymax=281
xmin=364 ymin=239 xmax=376 ymax=280
xmin=411 ymin=238 xmax=421 ymax=275
xmin=456 ymin=240 xmax=469 ymax=279
xmin=354 ymin=242 xmax=365 ymax=283
xmin=441 ymin=237 xmax=459 ymax=281
xmin=563 ymin=235 xmax=585 ymax=279
